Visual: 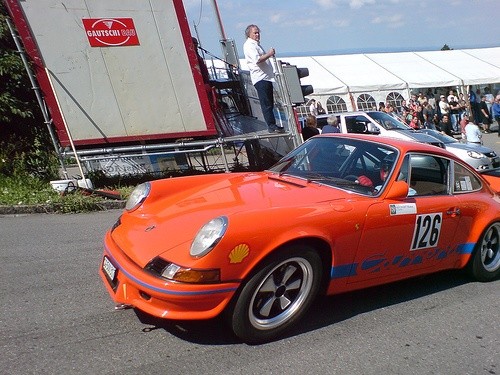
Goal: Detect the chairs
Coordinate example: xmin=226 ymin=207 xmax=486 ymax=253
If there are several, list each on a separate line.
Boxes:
xmin=409 ymin=167 xmax=448 ymax=196
xmin=350 ymin=168 xmax=376 ymax=184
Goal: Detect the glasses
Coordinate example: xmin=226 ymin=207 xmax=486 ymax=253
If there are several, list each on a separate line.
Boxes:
xmin=383 ymin=164 xmax=392 ymax=172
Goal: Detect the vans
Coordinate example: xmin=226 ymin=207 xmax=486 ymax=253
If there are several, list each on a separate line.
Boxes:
xmin=307 ymin=111 xmax=447 ymax=152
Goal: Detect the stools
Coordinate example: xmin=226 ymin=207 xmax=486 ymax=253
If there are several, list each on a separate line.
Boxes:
xmin=211 ymin=77 xmax=240 ymax=114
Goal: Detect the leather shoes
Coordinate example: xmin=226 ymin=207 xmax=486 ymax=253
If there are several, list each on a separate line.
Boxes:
xmin=269 ymin=128 xmax=285 ymax=133
xmin=268 ymin=127 xmax=284 ymax=132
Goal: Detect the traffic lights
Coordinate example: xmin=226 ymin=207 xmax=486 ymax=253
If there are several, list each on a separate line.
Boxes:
xmin=285 ymin=63 xmax=315 ymax=106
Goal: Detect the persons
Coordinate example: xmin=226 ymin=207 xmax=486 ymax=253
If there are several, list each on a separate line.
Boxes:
xmin=358 ymin=152 xmax=418 ymax=196
xmin=309 ymin=98 xmax=316 ymax=116
xmin=321 ymin=115 xmax=340 ymax=133
xmin=317 ymin=101 xmax=326 ymax=114
xmin=373 ymin=87 xmax=500 ymax=136
xmin=479 ymin=94 xmax=494 ymax=134
xmin=244 ymin=25 xmax=286 ymax=133
xmin=191 ymin=36 xmax=219 ymax=115
xmin=302 ymin=114 xmax=320 ymax=141
xmin=440 ymin=114 xmax=455 ymax=137
xmin=463 ymin=117 xmax=483 ymax=144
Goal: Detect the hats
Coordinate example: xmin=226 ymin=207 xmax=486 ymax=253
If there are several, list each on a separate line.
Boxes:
xmin=382 ymin=152 xmax=408 ymax=178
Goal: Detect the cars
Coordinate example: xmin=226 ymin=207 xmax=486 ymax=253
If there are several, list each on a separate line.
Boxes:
xmin=420 ymin=129 xmax=499 ymax=173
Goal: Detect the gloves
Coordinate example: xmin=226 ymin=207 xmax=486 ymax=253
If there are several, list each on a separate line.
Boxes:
xmin=355 ymin=175 xmax=374 ymax=192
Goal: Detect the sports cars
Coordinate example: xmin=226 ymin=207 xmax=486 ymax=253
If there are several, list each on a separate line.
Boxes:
xmin=97 ymin=133 xmax=499 ymax=345
